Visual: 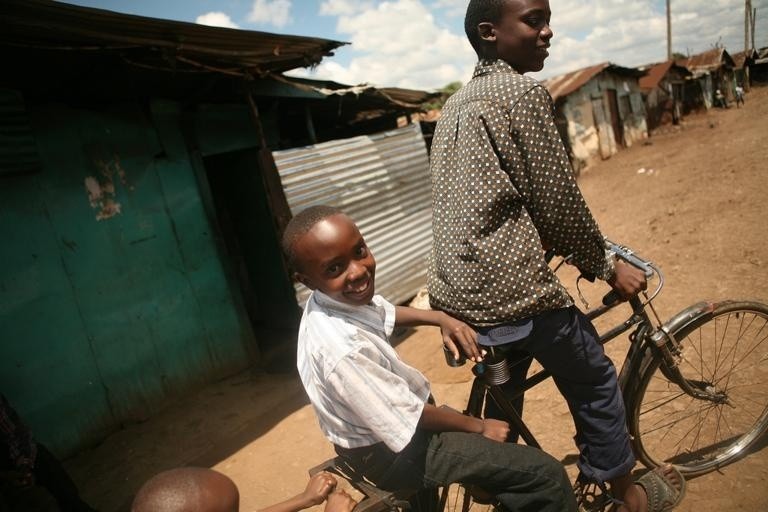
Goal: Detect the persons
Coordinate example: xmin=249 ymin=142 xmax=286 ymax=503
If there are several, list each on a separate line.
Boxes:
xmin=0 ymin=390 xmax=94 ymax=512
xmin=735 ymin=82 xmax=744 ymax=109
xmin=426 ymin=0 xmax=686 ymax=512
xmin=714 ymin=89 xmax=730 ymax=108
xmin=129 ymin=468 xmax=358 ymax=512
xmin=280 ymin=205 xmax=580 ymax=512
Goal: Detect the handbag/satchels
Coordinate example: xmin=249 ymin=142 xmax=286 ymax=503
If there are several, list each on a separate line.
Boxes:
xmin=354 ymin=447 xmax=426 ymax=501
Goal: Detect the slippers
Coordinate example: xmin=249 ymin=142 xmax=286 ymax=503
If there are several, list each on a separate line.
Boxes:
xmin=628 ymin=463 xmax=686 ymax=511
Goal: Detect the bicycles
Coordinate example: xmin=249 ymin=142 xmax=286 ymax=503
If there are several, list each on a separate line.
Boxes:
xmin=309 ymin=236 xmax=768 ymax=511
